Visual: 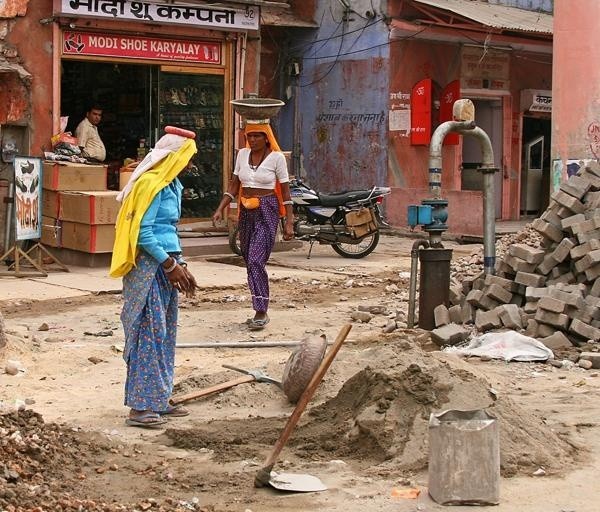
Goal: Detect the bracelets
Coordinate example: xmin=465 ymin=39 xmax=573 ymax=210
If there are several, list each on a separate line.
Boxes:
xmin=178 ymin=261 xmax=188 ymax=267
xmin=223 ymin=192 xmax=234 ymax=200
xmin=164 ymin=257 xmax=177 ymax=274
xmin=282 ymin=200 xmax=294 ymax=206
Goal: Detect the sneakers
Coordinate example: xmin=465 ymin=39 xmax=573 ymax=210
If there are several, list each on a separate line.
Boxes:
xmin=188 ymin=162 xmax=221 ymax=177
xmin=160 ymin=111 xmax=223 ymax=130
xmin=197 ymin=138 xmax=222 ymax=152
xmin=16 ymin=175 xmax=39 ymax=193
xmin=178 ymin=185 xmax=222 ymax=217
xmin=158 ymin=86 xmax=219 ymax=107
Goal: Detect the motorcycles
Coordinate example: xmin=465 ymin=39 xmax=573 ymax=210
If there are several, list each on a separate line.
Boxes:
xmin=228 ymin=177 xmax=391 ymax=259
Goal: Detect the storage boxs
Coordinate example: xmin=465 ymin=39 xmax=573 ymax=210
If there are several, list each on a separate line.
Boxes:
xmin=32 ymin=159 xmax=135 ymax=255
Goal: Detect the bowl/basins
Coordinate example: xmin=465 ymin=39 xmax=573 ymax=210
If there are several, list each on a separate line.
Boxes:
xmin=229 ymin=98 xmax=286 ymax=120
xmin=281 ymin=333 xmax=328 ymax=405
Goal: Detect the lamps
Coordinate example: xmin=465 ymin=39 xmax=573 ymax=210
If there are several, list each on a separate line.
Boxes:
xmin=40 ymin=17 xmax=76 ymax=30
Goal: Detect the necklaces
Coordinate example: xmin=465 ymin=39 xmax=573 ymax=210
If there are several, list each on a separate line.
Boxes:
xmin=250 ymin=150 xmax=266 ymax=172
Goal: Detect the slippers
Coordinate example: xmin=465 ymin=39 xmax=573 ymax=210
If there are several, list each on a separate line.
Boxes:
xmin=247 ymin=317 xmax=270 ymax=329
xmin=162 ymin=405 xmax=189 ymax=417
xmin=125 ymin=414 xmax=166 ymax=427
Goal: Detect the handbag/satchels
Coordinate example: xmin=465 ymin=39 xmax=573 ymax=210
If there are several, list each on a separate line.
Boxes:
xmin=346 ymin=207 xmax=377 ymax=239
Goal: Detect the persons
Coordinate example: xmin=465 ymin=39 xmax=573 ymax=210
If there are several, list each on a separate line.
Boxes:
xmin=74 ymin=103 xmax=107 ymax=164
xmin=109 ymin=124 xmax=200 ymax=429
xmin=210 ymin=118 xmax=296 ymax=328
